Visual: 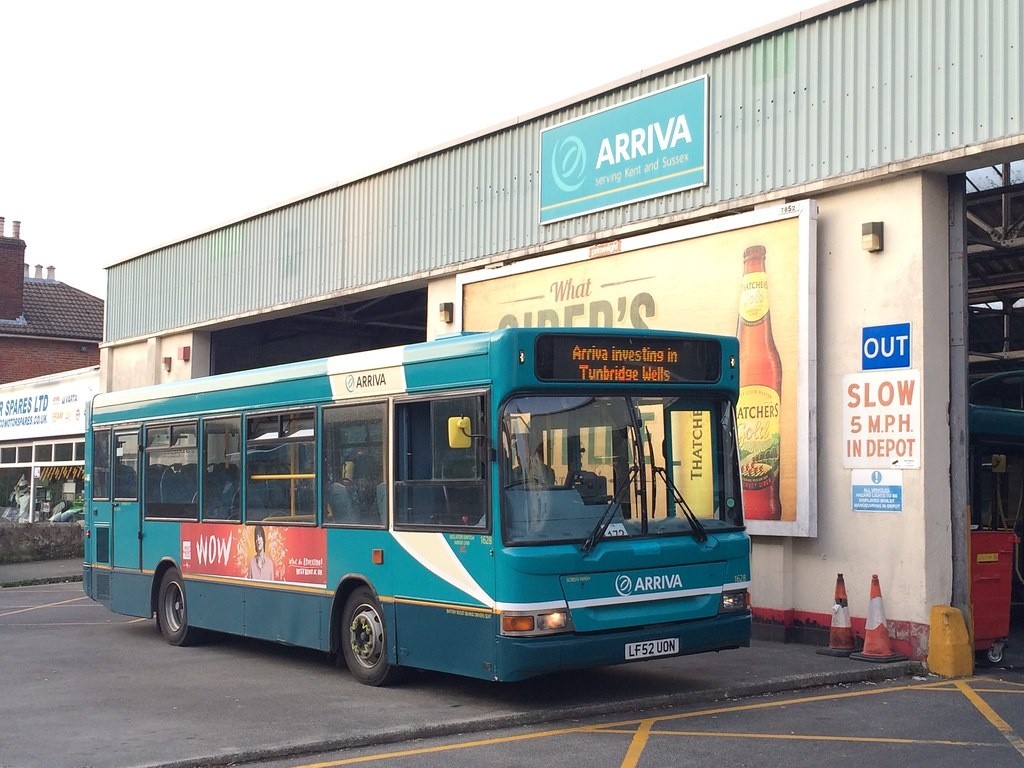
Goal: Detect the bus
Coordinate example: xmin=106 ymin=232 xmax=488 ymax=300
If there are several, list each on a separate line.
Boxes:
xmin=81 ymin=326 xmax=753 ymax=687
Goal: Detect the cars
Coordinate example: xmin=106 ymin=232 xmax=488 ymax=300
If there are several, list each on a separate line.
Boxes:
xmin=968 ymin=370 xmax=1024 ymax=457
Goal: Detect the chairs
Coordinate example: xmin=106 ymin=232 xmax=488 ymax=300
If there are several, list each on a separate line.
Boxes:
xmin=329 ymin=483 xmax=362 ymax=523
xmin=379 ymin=482 xmax=387 ymax=522
xmin=440 ymin=455 xmax=485 ymax=514
xmin=104 ymin=462 xmax=304 ymax=520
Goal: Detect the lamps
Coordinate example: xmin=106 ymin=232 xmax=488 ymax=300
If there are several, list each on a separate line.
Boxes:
xmin=439 ymin=302 xmax=453 ymax=324
xmin=161 ymin=357 xmax=171 ymax=372
xmin=860 ymin=221 xmax=884 ymax=254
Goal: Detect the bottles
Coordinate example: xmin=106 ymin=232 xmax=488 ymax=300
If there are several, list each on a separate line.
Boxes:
xmin=721 ymin=245 xmax=783 ymax=521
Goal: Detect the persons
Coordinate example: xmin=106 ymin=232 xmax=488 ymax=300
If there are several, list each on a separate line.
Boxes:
xmin=513 ymin=438 xmax=555 ymax=485
xmin=14 ymin=480 xmax=30 ymax=522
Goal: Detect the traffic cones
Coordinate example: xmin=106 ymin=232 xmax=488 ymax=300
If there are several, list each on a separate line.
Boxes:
xmin=848 ymin=574 xmax=910 ymax=663
xmin=815 ymin=573 xmax=862 ymax=658
